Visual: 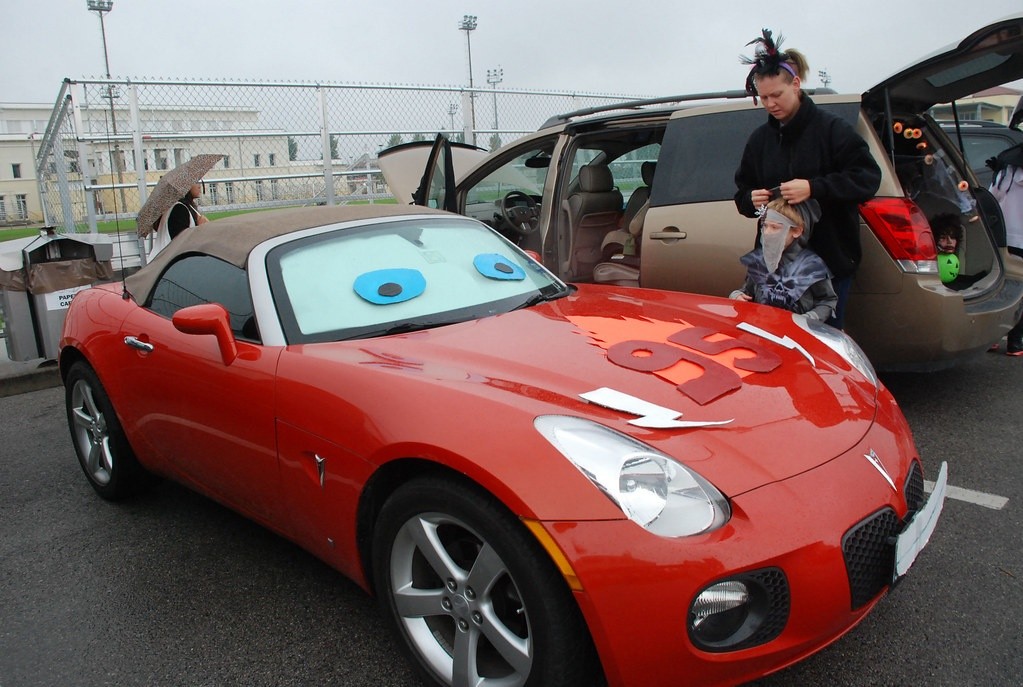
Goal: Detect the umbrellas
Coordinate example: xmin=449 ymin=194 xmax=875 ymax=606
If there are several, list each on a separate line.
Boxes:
xmin=138 ymin=154 xmax=224 ymax=241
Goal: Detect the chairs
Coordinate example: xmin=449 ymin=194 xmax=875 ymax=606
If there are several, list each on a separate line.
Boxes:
xmin=559 ymin=161 xmax=657 ymax=287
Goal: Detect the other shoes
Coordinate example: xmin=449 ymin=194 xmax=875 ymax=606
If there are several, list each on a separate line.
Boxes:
xmin=1007 ymin=345 xmax=1023 ymax=355
xmin=988 ymin=343 xmax=998 ymax=352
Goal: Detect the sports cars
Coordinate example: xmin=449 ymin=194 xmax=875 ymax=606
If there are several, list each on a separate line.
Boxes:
xmin=58 ymin=202 xmax=946 ymax=687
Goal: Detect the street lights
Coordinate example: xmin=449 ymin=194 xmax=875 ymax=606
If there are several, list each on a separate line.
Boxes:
xmin=486 ymin=68 xmax=504 ymax=137
xmin=448 ymin=103 xmax=459 ymax=141
xmin=87 ymin=0 xmax=130 ymax=216
xmin=459 ymin=15 xmax=478 ymax=148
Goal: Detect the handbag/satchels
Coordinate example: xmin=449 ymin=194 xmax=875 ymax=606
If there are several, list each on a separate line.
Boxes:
xmin=146 ymin=202 xmax=197 ymax=263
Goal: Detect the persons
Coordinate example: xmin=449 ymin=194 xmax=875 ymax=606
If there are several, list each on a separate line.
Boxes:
xmin=729 ymin=193 xmax=840 ymax=323
xmin=984 ymin=141 xmax=1023 ymax=356
xmin=147 ymin=181 xmax=210 ymax=264
xmin=927 ymin=212 xmax=964 ymax=253
xmin=735 ymin=48 xmax=883 ymax=336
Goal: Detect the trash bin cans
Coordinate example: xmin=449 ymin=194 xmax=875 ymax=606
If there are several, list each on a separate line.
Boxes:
xmin=0 ymin=224 xmax=117 ymax=362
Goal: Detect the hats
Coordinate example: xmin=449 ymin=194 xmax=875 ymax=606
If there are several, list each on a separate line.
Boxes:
xmin=767 ymin=186 xmax=823 ymax=244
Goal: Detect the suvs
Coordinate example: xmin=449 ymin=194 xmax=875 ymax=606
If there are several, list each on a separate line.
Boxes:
xmin=930 ymin=94 xmax=1023 ymax=192
xmin=374 ymin=11 xmax=1023 ymax=379
